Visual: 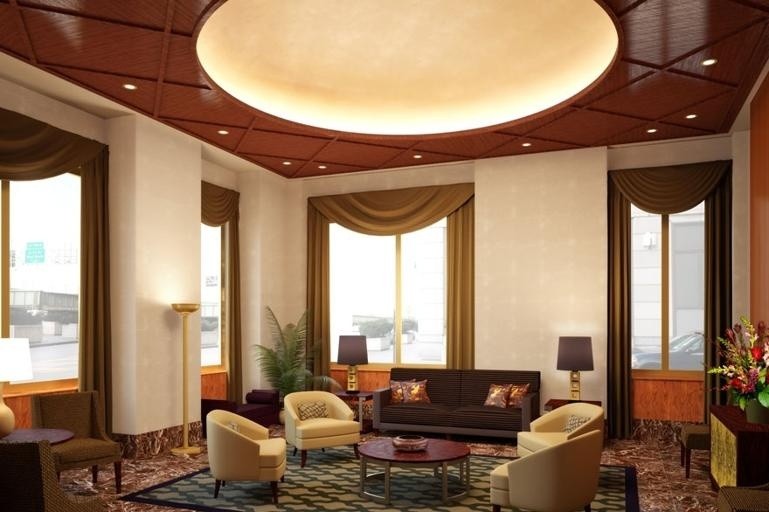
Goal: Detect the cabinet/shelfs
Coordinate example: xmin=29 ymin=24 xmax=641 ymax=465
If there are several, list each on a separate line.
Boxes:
xmin=706 ymin=408 xmax=769 ymax=489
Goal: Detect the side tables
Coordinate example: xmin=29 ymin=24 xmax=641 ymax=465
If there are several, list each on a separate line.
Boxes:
xmin=543 ymin=399 xmax=602 ymax=413
xmin=335 ymin=390 xmax=373 ymax=434
xmin=0 ymin=429 xmax=73 ymax=446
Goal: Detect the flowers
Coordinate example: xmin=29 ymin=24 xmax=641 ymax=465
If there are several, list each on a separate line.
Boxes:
xmin=695 ymin=317 xmax=769 ymax=407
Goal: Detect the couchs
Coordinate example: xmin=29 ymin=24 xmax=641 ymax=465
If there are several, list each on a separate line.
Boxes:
xmin=372 ymin=367 xmax=540 ymax=443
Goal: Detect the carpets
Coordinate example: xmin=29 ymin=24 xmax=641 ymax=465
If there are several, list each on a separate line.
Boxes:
xmin=117 ymin=447 xmax=638 ymax=512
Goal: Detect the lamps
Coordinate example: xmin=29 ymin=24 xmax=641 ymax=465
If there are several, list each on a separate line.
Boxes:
xmin=192 ymin=2 xmax=619 ymax=138
xmin=337 ymin=335 xmax=368 ymax=393
xmin=522 ymin=143 xmax=531 ymax=146
xmin=0 ymin=336 xmax=30 ymax=439
xmin=647 ymin=129 xmax=657 ymax=132
xmin=124 ymin=85 xmax=135 ymax=89
xmin=171 ymin=303 xmax=201 ymax=455
xmin=687 ymin=114 xmax=697 ymax=118
xmin=414 ymin=155 xmax=422 ymax=158
xmin=556 ymin=337 xmax=594 ymax=401
xmin=319 ymin=166 xmax=326 ymax=169
xmin=704 ymin=60 xmax=716 ymax=66
xmin=218 ymin=130 xmax=229 ymax=135
xmin=283 ymin=162 xmax=291 ymax=165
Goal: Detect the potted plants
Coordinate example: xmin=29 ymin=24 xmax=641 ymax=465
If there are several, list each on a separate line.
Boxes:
xmin=252 ymin=305 xmax=343 ymax=424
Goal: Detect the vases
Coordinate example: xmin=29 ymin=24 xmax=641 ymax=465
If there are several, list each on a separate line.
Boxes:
xmin=743 ymin=398 xmax=766 ymax=424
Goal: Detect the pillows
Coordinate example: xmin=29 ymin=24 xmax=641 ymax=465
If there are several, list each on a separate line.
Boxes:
xmin=399 ymin=380 xmax=432 ymax=404
xmin=484 ymin=383 xmax=513 ymax=409
xmin=508 ymin=383 xmax=530 ymax=408
xmin=388 ymin=379 xmax=416 ymax=405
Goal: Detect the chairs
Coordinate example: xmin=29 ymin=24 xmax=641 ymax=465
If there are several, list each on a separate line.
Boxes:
xmin=715 ymin=485 xmax=768 ymax=512
xmin=0 ymin=441 xmax=108 ymax=512
xmin=205 ymin=410 xmax=287 ymax=500
xmin=681 ymin=423 xmax=711 ymax=479
xmin=489 ymin=430 xmax=601 ymax=512
xmin=517 ymin=404 xmax=606 ymax=456
xmin=31 ymin=391 xmax=124 ymax=495
xmin=283 ymin=391 xmax=364 ymax=466
